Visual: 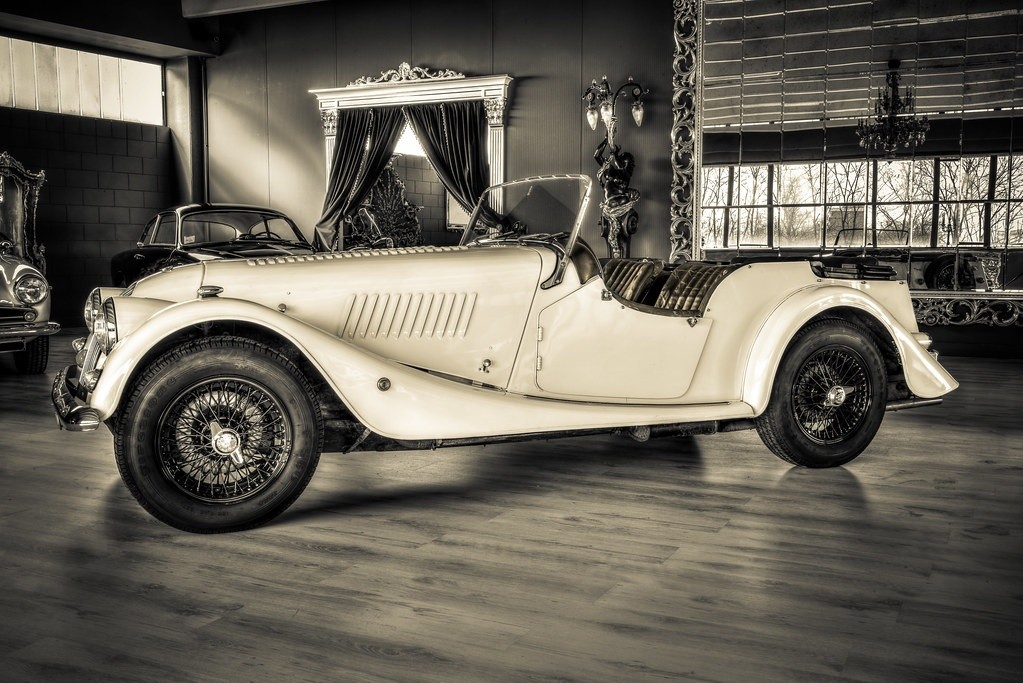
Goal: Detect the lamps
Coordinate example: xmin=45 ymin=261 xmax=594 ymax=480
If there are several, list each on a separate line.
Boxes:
xmin=582 ymin=73 xmax=653 ymax=258
xmin=854 ymin=39 xmax=933 ymax=166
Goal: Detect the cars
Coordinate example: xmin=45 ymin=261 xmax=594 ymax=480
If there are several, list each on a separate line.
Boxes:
xmin=111 ymin=203 xmax=322 ymax=290
xmin=0 ymin=151 xmax=60 ymax=377
xmin=52 ymin=172 xmax=963 ymax=534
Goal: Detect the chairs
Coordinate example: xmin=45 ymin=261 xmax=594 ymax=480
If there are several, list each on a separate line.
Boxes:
xmin=598 ymin=259 xmax=737 ymax=313
